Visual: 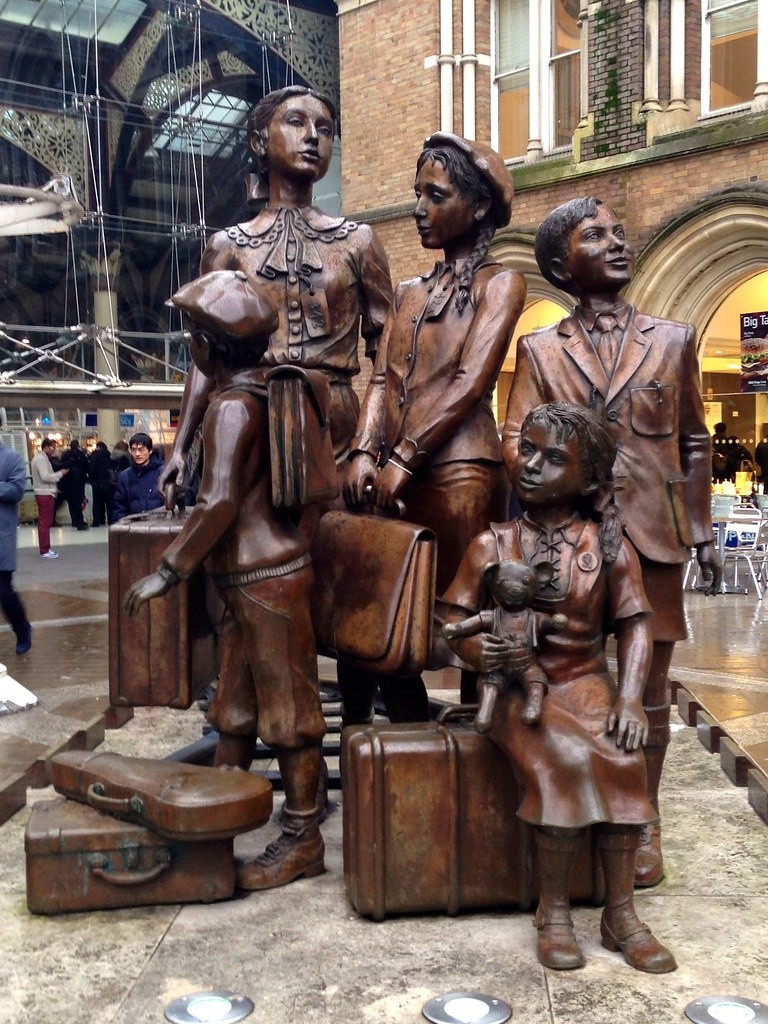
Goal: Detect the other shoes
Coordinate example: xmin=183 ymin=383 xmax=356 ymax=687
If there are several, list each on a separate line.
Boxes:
xmin=14 ymin=619 xmax=32 ymax=655
xmin=40 ymin=549 xmax=59 ymax=560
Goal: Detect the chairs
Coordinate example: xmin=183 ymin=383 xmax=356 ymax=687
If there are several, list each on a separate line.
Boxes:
xmin=682 ymin=493 xmax=741 ymax=589
xmin=705 ymin=507 xmax=764 ymax=601
xmin=730 ymin=495 xmax=768 ymax=594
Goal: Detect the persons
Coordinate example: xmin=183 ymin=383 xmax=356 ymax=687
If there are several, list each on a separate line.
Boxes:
xmin=0 ymin=415 xmax=31 ymax=654
xmin=113 ymin=432 xmax=165 ymax=522
xmin=32 ymin=438 xmax=69 ymax=559
xmin=345 ymin=132 xmax=526 ymax=728
xmin=503 ymin=199 xmax=725 ymax=888
xmin=110 ymin=443 xmax=132 ymax=472
xmin=155 ymin=86 xmax=396 ymax=825
xmin=439 ymin=404 xmax=677 ymax=973
xmin=60 ymin=441 xmax=89 ymax=531
xmin=121 ymin=272 xmax=324 ymax=890
xmin=755 ymin=435 xmax=768 ymax=494
xmin=710 ymin=422 xmax=730 ymax=484
xmin=88 ymin=443 xmax=115 ymax=526
xmin=726 ymin=437 xmax=753 ymax=483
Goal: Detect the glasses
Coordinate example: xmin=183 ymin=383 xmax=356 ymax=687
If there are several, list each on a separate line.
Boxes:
xmin=130 ymin=446 xmax=147 ymax=454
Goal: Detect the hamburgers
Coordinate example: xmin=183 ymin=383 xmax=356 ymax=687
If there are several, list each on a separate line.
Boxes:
xmin=740 ymin=338 xmax=768 ymax=378
